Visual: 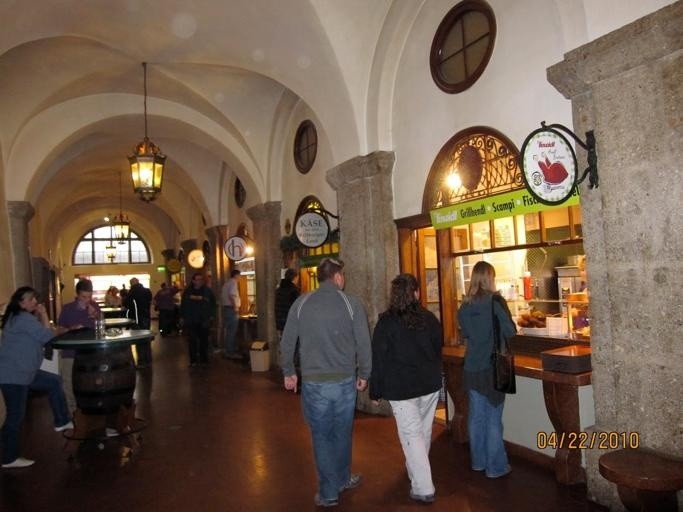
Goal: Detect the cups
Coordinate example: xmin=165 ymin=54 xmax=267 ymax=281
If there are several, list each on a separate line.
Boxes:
xmin=95 ymin=319 xmax=105 ymax=331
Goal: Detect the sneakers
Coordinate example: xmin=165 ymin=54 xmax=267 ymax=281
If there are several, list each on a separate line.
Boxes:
xmin=486 ymin=463 xmax=516 ymax=480
xmin=471 ymin=463 xmax=485 ymax=471
xmin=311 ymin=491 xmax=340 ymax=507
xmin=344 ymin=472 xmax=368 ymax=490
xmin=52 ymin=421 xmax=74 ymax=431
xmin=0 ymin=457 xmax=37 ymax=467
xmin=407 ymin=487 xmax=435 ymax=504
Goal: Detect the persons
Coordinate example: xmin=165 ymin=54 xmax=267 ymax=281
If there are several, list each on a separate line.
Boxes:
xmin=458 ymin=262 xmax=517 ymax=478
xmin=56 ymin=280 xmax=102 ymax=415
xmin=277 ymin=257 xmax=373 ymax=506
xmin=275 ymin=270 xmax=300 ymax=388
xmin=1 ymin=286 xmax=73 ymax=469
xmin=370 ymin=274 xmax=445 ymax=503
xmin=104 ymin=269 xmax=241 ymax=368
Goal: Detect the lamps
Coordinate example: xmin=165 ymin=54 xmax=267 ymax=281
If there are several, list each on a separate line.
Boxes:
xmin=103 ymin=213 xmax=112 ymax=222
xmin=127 ymin=61 xmax=168 ymax=203
xmin=114 ymin=171 xmax=130 ymax=244
xmin=106 ymin=224 xmax=116 ymax=258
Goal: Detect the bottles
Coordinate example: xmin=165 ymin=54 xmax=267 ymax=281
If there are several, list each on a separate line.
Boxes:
xmin=523 ymin=271 xmax=532 ymax=300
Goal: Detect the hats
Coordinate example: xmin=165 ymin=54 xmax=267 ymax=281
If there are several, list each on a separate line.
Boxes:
xmin=316 ymin=255 xmax=346 ymax=279
xmin=284 ymin=268 xmax=298 ymax=280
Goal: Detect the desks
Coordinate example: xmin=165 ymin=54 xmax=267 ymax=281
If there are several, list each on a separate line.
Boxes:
xmin=104 ymin=318 xmax=136 ymax=330
xmin=51 ymin=328 xmax=155 ymax=464
xmin=95 ymin=300 xmax=124 ymax=317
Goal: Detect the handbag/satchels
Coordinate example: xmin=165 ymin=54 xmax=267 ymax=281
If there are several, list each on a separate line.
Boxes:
xmin=488 ymin=351 xmax=520 ymax=393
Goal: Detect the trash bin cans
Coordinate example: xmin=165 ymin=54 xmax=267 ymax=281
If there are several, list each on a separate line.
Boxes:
xmin=248 ymin=340 xmax=271 ymax=372
